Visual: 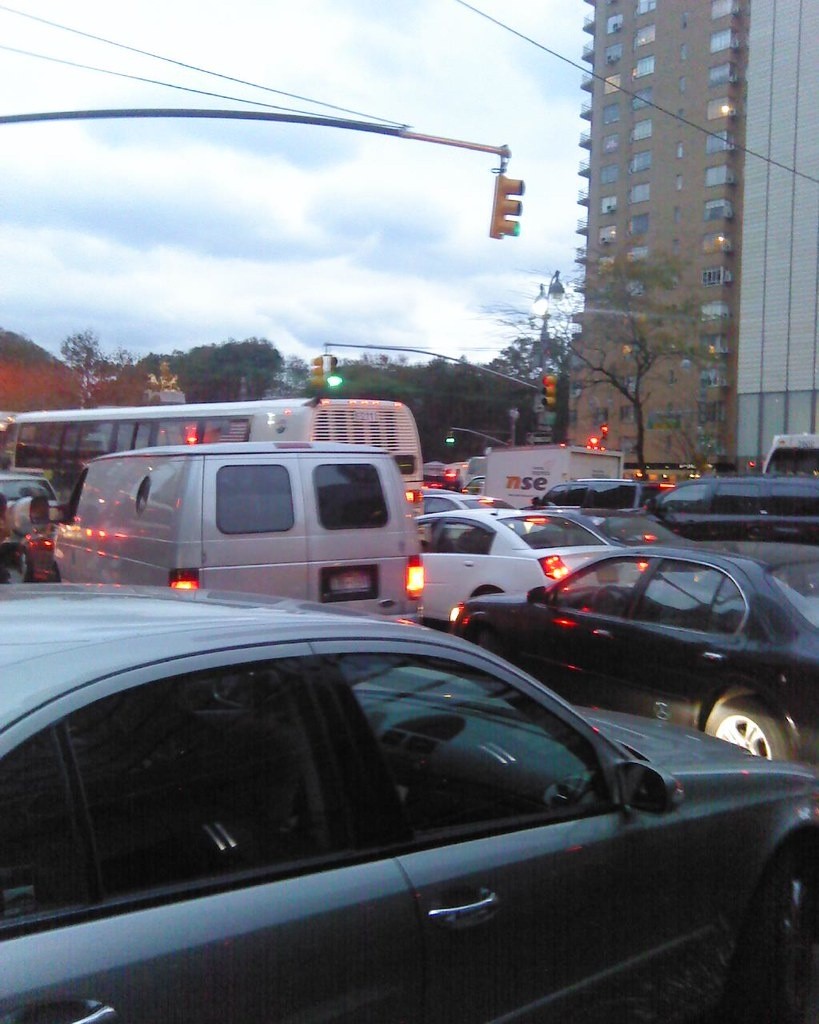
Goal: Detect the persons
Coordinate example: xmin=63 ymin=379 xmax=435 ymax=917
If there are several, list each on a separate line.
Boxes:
xmin=0 ymin=492 xmax=11 ymax=584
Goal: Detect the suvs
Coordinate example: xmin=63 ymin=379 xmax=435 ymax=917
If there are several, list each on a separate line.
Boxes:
xmin=0 ymin=471 xmax=61 ymax=558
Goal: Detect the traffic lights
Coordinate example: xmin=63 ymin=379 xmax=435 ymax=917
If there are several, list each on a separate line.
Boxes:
xmin=327 ymin=375 xmax=343 ymax=388
xmin=489 ymin=175 xmax=524 ymax=239
xmin=542 ymin=376 xmax=556 ymax=406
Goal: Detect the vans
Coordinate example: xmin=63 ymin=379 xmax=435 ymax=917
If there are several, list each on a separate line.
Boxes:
xmin=49 ymin=441 xmax=424 ymax=623
xmin=520 ymin=476 xmax=819 ymax=543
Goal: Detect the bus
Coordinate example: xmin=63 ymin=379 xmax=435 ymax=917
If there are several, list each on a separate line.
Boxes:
xmin=0 ymin=398 xmax=424 ymax=518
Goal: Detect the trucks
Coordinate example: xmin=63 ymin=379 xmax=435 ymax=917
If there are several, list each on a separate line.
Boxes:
xmin=459 ymin=445 xmax=624 ymax=508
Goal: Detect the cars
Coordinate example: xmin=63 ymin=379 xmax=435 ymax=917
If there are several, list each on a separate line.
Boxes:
xmin=15 ymin=501 xmax=69 ymax=582
xmin=413 ymin=488 xmax=819 ymax=762
xmin=1 ymin=585 xmax=819 ymax=1023
